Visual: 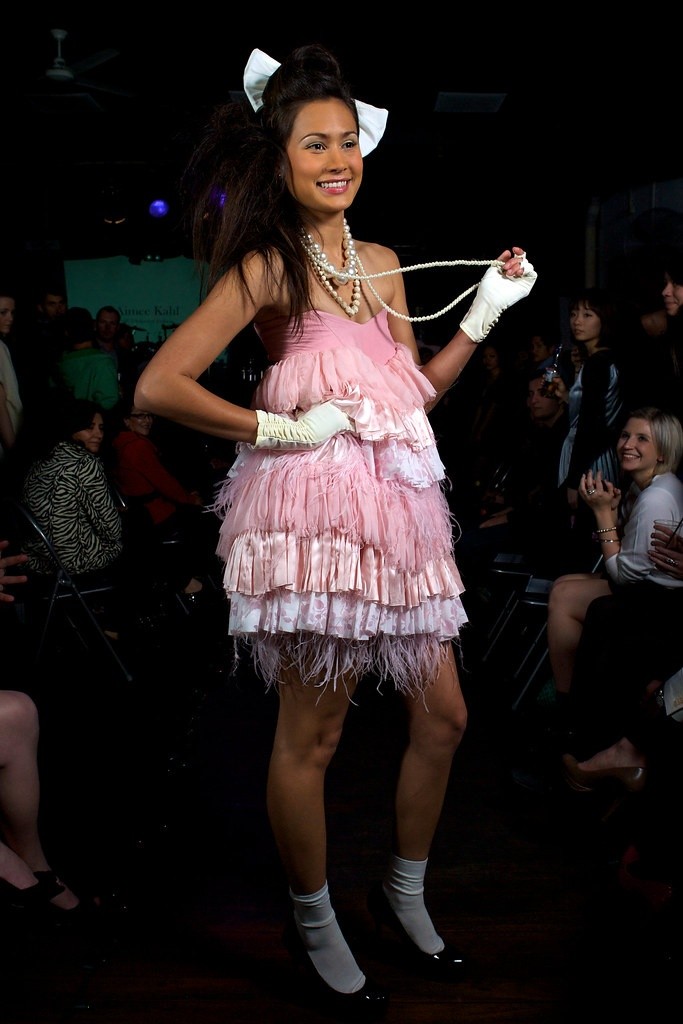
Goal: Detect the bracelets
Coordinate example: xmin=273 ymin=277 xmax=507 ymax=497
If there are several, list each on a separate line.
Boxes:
xmin=597 ymin=526 xmax=616 ymax=532
xmin=597 ymin=538 xmax=620 ymax=542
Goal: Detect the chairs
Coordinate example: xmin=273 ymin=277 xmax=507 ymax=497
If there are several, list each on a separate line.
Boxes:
xmin=480 ymin=542 xmax=603 ymax=712
xmin=4 ymin=480 xmax=189 ymax=682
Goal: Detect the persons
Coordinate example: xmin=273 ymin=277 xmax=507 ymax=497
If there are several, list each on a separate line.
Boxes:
xmin=134 ymin=43 xmax=538 ymax=1017
xmin=470 ymin=247 xmax=683 ymax=821
xmin=0 ymin=285 xmax=216 ymax=929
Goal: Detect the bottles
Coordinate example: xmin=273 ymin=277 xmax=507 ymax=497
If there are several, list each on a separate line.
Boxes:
xmin=541 ymin=344 xmax=564 ymax=398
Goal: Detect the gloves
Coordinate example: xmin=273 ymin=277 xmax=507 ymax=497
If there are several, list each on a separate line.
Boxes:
xmin=248 ymin=399 xmax=360 ymax=452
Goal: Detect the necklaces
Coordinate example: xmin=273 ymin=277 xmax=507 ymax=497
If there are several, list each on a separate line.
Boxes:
xmin=299 ymin=217 xmax=506 ymax=322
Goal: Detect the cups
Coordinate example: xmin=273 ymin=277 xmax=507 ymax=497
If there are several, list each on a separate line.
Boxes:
xmin=654 ymin=519 xmax=683 ymax=570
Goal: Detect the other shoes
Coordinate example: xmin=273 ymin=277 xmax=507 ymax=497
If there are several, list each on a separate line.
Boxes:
xmin=362 ymin=879 xmax=463 ymax=985
xmin=289 ymin=909 xmax=395 ymax=1018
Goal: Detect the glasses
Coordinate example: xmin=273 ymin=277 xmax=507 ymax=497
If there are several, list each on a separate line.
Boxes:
xmin=129 ymin=413 xmax=154 ymax=420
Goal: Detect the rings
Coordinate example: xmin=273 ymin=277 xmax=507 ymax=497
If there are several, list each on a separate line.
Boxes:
xmin=666 ymin=557 xmax=678 ymax=566
xmin=587 ymin=489 xmax=595 ymax=495
